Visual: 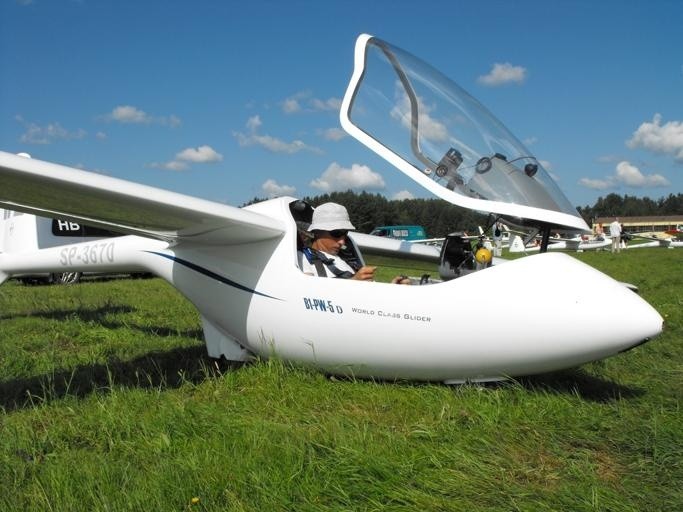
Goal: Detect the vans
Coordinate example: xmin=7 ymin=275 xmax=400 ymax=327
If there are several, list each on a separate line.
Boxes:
xmin=368 ymin=225 xmax=426 ymax=240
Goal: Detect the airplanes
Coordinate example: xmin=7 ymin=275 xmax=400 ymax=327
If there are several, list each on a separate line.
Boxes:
xmin=408 ymin=224 xmax=682 ymax=260
xmin=0 ymin=32 xmax=665 ymax=384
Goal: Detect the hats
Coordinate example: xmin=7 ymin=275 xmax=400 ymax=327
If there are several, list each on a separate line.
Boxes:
xmin=307 ymin=202 xmax=357 ymax=232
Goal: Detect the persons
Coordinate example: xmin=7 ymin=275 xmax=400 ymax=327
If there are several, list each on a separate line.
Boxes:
xmin=534 ymin=234 xmax=541 ymax=248
xmin=553 ymin=232 xmax=561 ymax=239
xmin=490 ymin=222 xmax=504 ymax=258
xmin=295 ymin=202 xmax=412 ymax=284
xmin=462 ymin=229 xmax=472 ymax=237
xmin=608 ymin=217 xmax=622 ymax=254
xmin=595 ymin=222 xmax=608 ymax=251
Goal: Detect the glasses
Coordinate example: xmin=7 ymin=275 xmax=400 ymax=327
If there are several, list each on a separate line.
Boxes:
xmin=326 ymin=230 xmax=348 ymax=238
